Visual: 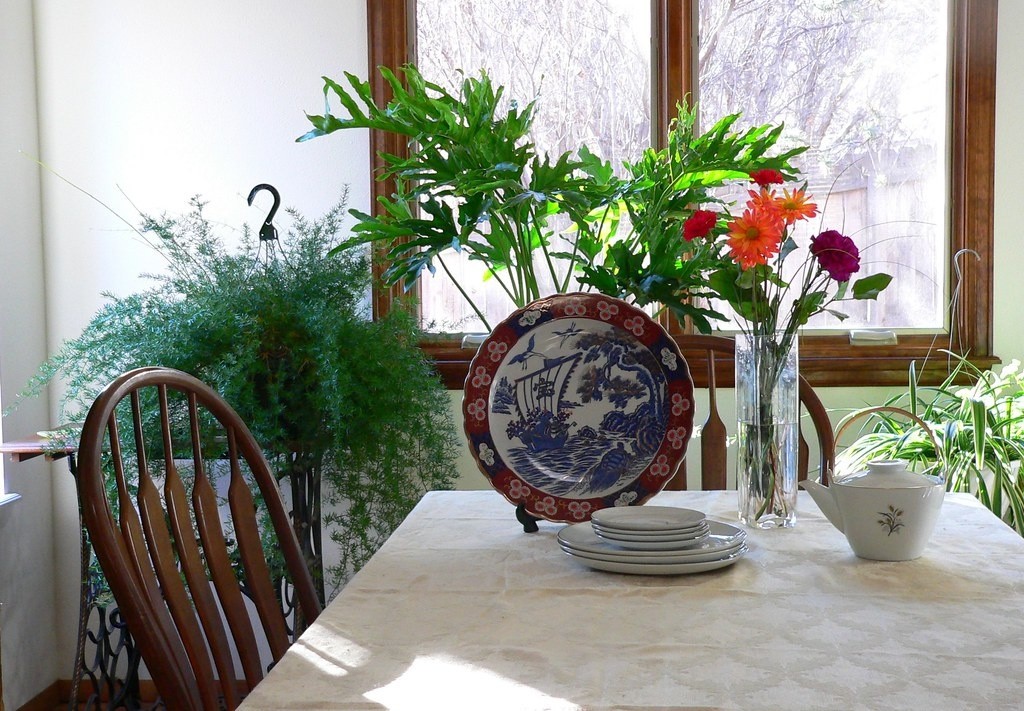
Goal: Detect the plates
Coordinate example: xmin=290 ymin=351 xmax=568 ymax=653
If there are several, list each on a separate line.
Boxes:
xmin=556 ymin=506 xmax=748 ymax=576
xmin=462 ymin=292 xmax=695 ymax=525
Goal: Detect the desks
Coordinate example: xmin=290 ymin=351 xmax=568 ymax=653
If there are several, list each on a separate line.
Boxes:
xmin=242 ymin=491 xmax=1024 ymax=711
xmin=0 ymin=433 xmax=331 ymax=711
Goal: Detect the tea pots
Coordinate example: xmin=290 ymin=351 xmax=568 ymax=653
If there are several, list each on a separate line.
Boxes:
xmin=798 ymin=407 xmax=947 ymax=562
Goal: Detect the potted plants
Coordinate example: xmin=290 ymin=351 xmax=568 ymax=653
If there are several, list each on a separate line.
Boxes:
xmin=835 ymin=355 xmax=1024 ymax=540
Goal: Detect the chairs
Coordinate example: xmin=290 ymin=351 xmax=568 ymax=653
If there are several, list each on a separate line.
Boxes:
xmin=663 ymin=335 xmax=836 ymax=491
xmin=77 ymin=364 xmax=325 ymax=711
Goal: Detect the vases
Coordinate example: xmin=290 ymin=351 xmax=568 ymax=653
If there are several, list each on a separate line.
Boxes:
xmin=678 ymin=168 xmax=892 ymax=331
xmin=731 ymin=331 xmax=801 ymax=533
